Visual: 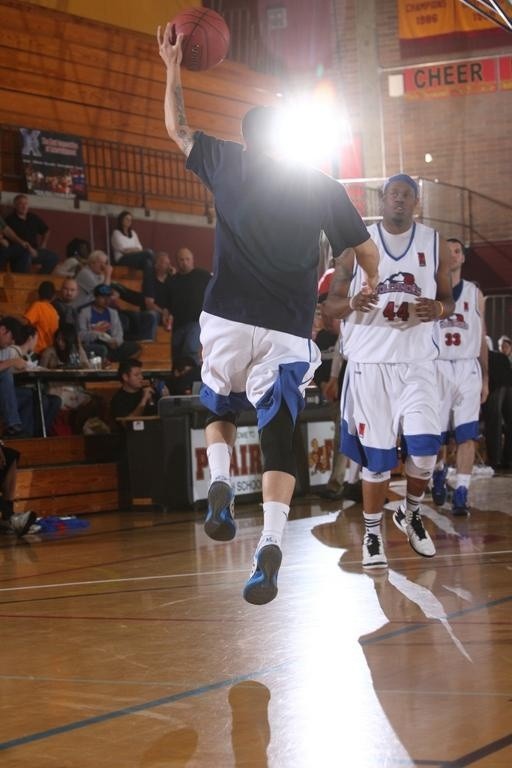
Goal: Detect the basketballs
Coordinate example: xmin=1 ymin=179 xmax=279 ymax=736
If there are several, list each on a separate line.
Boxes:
xmin=171 ymin=6 xmax=230 ymax=72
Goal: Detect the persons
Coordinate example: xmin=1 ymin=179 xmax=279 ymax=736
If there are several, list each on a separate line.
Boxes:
xmin=312 ymin=175 xmax=512 ymax=565
xmin=0 ymin=195 xmax=212 ymax=442
xmin=156 ymin=21 xmax=379 ymax=604
xmin=0 ymin=445 xmax=36 ymax=537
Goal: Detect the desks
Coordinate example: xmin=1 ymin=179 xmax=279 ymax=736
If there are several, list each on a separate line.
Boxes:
xmin=156 ymin=386 xmax=341 ymax=512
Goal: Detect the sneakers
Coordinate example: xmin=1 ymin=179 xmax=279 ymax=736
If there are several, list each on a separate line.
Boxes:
xmin=431 ymin=464 xmax=449 ymax=506
xmin=0 ymin=510 xmax=42 ymax=538
xmin=451 ymin=485 xmax=470 ymax=517
xmin=361 ymin=531 xmax=389 ymax=570
xmin=3 ymin=427 xmax=31 ymax=438
xmin=243 ymin=535 xmax=282 ymax=606
xmin=204 ymin=480 xmax=236 ymax=542
xmin=320 ymin=489 xmax=343 ymax=501
xmin=392 ymin=504 xmax=437 ymax=558
xmin=343 ymin=479 xmax=389 ymax=506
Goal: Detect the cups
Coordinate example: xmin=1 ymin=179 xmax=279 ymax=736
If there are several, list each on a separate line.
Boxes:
xmin=165 ymin=319 xmax=172 ymax=330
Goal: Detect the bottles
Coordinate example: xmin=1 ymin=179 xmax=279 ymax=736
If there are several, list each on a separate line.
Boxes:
xmin=47 ymin=344 xmax=102 ymax=369
